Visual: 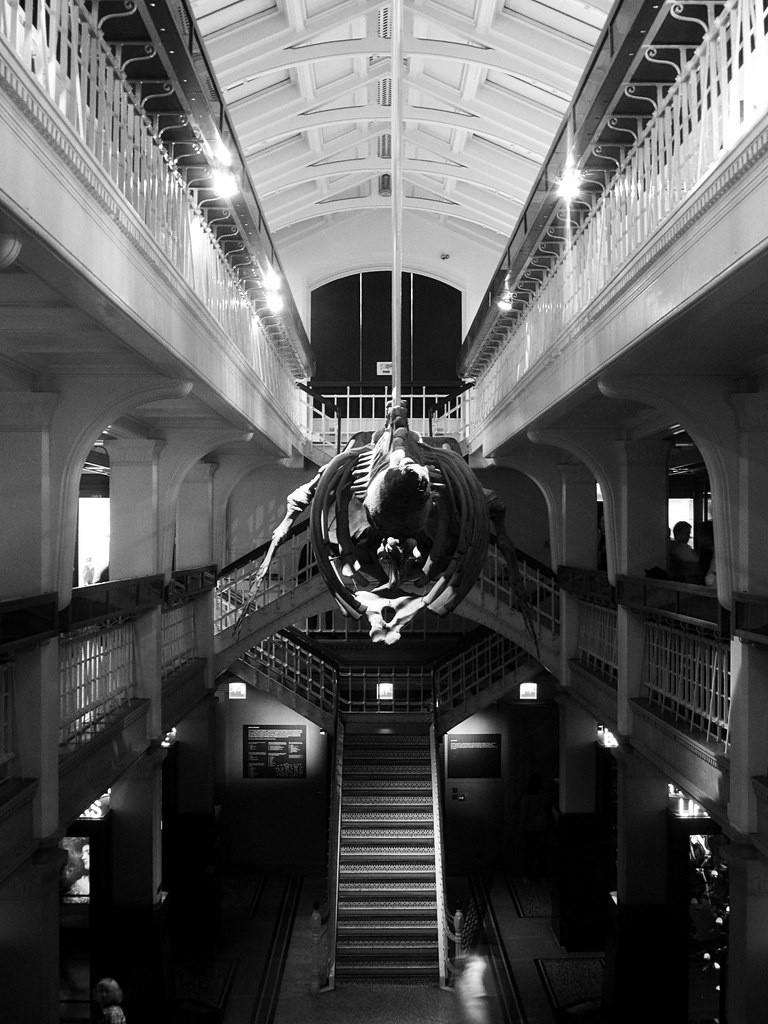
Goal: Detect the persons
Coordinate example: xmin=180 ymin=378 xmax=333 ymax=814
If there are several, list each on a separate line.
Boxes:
xmin=94 ymin=979 xmax=125 ymax=1024
xmin=64 ymin=840 xmax=90 ymax=903
xmin=518 ymin=773 xmax=552 ymax=882
xmin=688 ymin=836 xmax=730 ymax=975
xmin=668 ymin=519 xmax=715 ymax=587
